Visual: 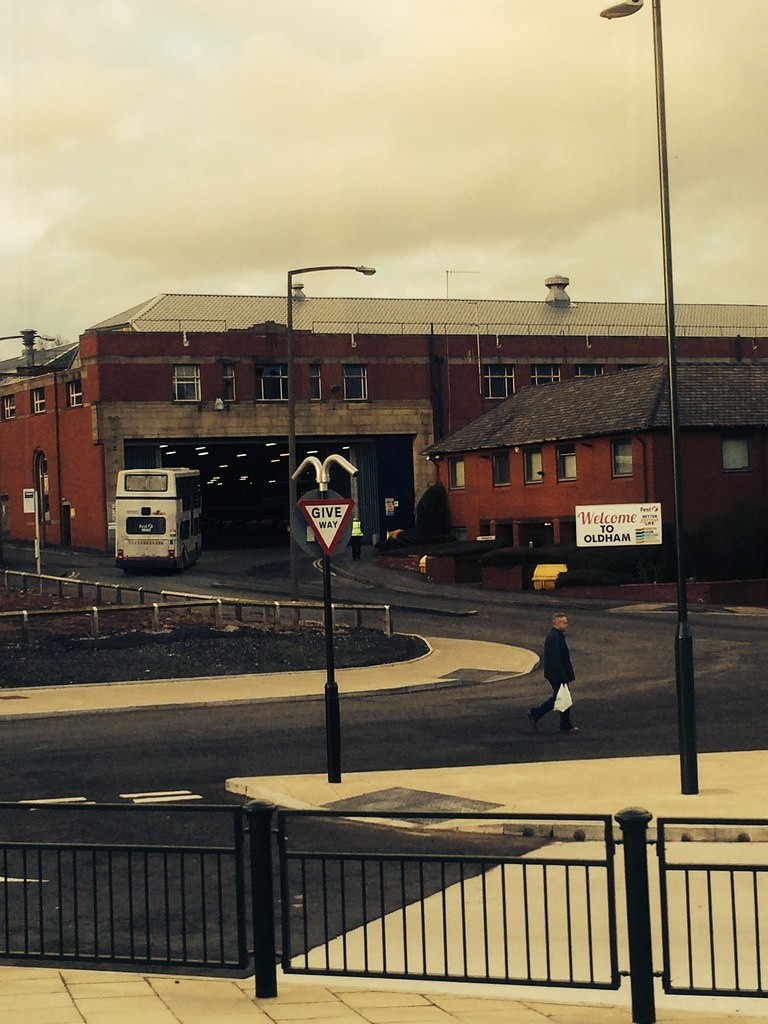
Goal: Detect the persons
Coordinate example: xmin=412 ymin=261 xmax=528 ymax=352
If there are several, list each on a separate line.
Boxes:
xmin=527 ymin=613 xmax=580 ymax=734
xmin=350 ymin=517 xmax=363 ymax=560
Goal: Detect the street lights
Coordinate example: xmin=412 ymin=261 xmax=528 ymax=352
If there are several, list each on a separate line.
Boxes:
xmin=604 ymin=2 xmax=699 ymax=796
xmin=287 ymin=264 xmax=379 ymax=602
xmin=291 ymin=455 xmax=359 ymax=782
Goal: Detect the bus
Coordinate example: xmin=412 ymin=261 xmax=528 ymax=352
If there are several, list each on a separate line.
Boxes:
xmin=113 ymin=468 xmax=205 ymax=576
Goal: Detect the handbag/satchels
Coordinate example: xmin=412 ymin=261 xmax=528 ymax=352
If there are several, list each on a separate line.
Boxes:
xmin=554 ymin=682 xmax=574 ymax=712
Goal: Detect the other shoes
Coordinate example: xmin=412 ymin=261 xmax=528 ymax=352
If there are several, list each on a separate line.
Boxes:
xmin=353 ymin=555 xmax=360 ymax=560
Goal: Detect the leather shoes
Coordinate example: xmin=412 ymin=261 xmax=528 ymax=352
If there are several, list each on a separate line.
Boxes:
xmin=560 ymin=726 xmax=582 ymax=734
xmin=527 ymin=709 xmax=537 ymax=730
